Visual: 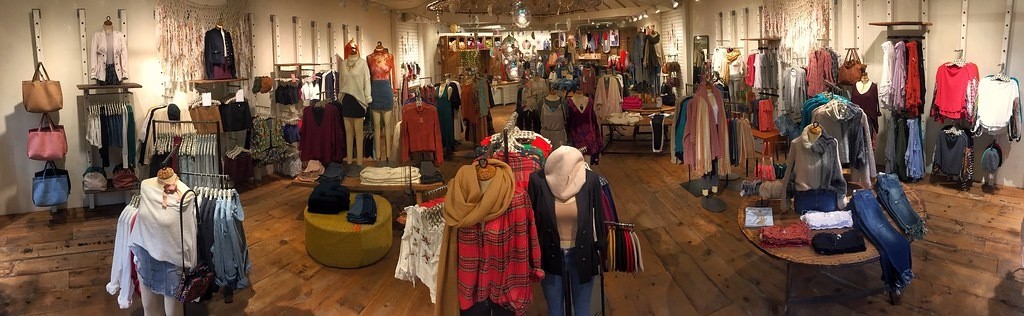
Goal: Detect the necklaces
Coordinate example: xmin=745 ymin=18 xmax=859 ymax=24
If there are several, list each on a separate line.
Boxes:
xmin=574 ymin=94 xmax=586 ymax=110
xmin=346 ymin=58 xmax=360 ymax=72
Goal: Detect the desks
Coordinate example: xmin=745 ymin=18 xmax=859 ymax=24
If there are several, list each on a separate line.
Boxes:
xmin=600 ymin=114 xmax=674 ymax=154
xmin=738 ymin=168 xmax=926 ymax=316
xmin=292 ymin=171 xmax=443 ymax=220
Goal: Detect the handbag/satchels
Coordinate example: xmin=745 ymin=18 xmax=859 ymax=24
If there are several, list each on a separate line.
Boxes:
xmin=175 ymin=261 xmax=214 ymax=303
xmin=22 ymin=62 xmax=63 ymax=112
xmin=32 ymin=161 xmax=71 ymax=207
xmin=26 ymin=113 xmax=68 ymax=160
xmin=838 ymin=48 xmax=867 ymax=84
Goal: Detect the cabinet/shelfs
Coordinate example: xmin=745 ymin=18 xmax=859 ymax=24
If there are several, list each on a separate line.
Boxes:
xmin=440 ymin=36 xmax=502 ymax=84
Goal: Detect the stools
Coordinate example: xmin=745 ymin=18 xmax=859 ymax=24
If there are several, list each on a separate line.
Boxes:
xmin=304 ymin=192 xmax=393 ymax=268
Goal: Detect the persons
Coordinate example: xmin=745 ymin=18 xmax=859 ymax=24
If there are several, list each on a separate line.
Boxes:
xmin=851 ymin=73 xmax=882 ymax=133
xmin=781 ymin=121 xmax=847 ymax=215
xmin=537 ymin=90 xmax=567 ymax=150
xmin=537 ymin=56 xmax=544 ymax=78
xmin=366 ymin=41 xmax=398 ymax=163
xmin=682 ymin=81 xmax=731 ymax=178
xmin=521 ymin=61 xmax=533 ymax=85
xmin=338 ymin=37 xmax=372 ymax=166
xmin=434 ymin=146 xmax=608 ymax=316
xmin=90 ymin=20 xmax=129 ymax=86
xmin=643 ymin=30 xmax=662 ymax=68
xmin=462 ymin=78 xmax=480 ymax=124
xmin=127 ymin=168 xmax=198 ymax=316
xmin=434 ymin=82 xmax=452 ymax=128
xmin=529 ymin=73 xmax=546 ymax=97
xmin=548 ymin=66 xmax=559 ymax=90
xmin=205 ymin=20 xmax=237 ymax=79
xmin=581 ymin=61 xmax=597 ymax=98
xmin=567 ymin=89 xmax=603 ymax=155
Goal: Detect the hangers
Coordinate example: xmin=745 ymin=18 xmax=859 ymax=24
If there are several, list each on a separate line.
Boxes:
xmin=118 ymin=172 xmax=238 ymax=223
xmin=304 ymin=90 xmax=340 ymax=110
xmin=400 ymin=185 xmax=447 ymax=238
xmin=940 ymin=120 xmax=967 ymax=141
xmin=88 ymin=100 xmax=133 ymax=116
xmin=476 ymin=112 xmax=552 ymax=168
xmin=980 ymin=63 xmax=1019 ymax=85
xmin=938 ymin=50 xmax=977 ymax=74
xmin=187 ymin=87 xmax=249 ymax=109
xmin=224 ymin=138 xmax=252 ymax=160
xmin=154 ymin=120 xmax=218 ymax=157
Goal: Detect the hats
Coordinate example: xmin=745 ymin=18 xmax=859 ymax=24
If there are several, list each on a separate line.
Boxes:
xmin=545 ymin=145 xmax=586 ymax=203
